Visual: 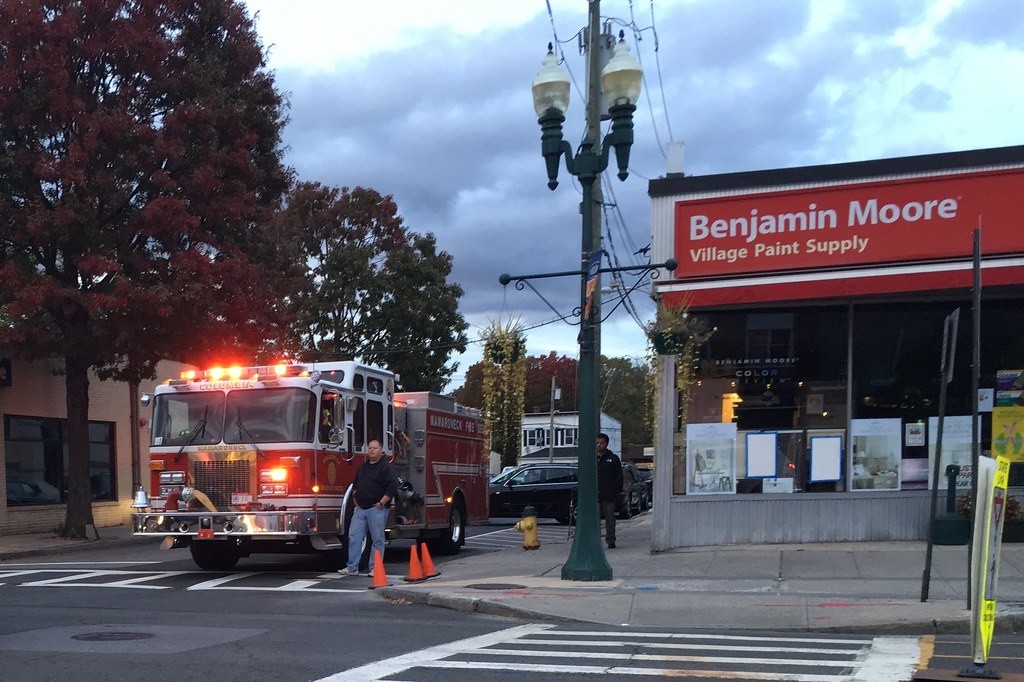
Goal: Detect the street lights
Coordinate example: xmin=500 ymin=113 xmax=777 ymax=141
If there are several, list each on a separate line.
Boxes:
xmin=531 ymin=30 xmax=642 ymax=583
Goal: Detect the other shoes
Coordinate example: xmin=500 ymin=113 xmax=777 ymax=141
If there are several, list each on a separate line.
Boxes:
xmin=608 ymin=542 xmax=615 ymax=548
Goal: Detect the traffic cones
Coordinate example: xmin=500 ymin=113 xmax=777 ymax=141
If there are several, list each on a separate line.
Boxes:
xmin=404 ymin=545 xmax=427 ymax=581
xmin=369 ymin=549 xmax=394 ymax=588
xmin=421 ymin=542 xmax=440 ymax=577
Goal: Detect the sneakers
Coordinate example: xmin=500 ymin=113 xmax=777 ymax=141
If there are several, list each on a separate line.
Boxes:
xmin=367 ymin=569 xmax=374 ymax=576
xmin=338 ymin=568 xmax=359 ymax=576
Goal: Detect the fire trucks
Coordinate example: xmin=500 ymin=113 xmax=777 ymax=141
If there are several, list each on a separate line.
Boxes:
xmin=130 ymin=360 xmax=490 ymax=571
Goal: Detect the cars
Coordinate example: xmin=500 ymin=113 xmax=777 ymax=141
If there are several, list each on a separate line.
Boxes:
xmin=63 ymin=472 xmax=115 ymax=502
xmin=488 ymin=461 xmax=653 ymax=525
xmin=6 ymin=477 xmax=59 ymax=504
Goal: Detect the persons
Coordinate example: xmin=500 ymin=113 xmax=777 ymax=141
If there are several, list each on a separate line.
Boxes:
xmin=179 ymin=406 xmax=220 ymax=445
xmin=338 ymin=440 xmax=398 ymax=578
xmin=594 ymin=433 xmax=626 ymax=549
xmin=290 ymin=399 xmax=331 ymax=443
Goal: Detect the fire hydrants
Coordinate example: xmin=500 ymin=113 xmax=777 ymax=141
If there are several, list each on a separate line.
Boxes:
xmin=515 ymin=506 xmax=541 ymax=550
